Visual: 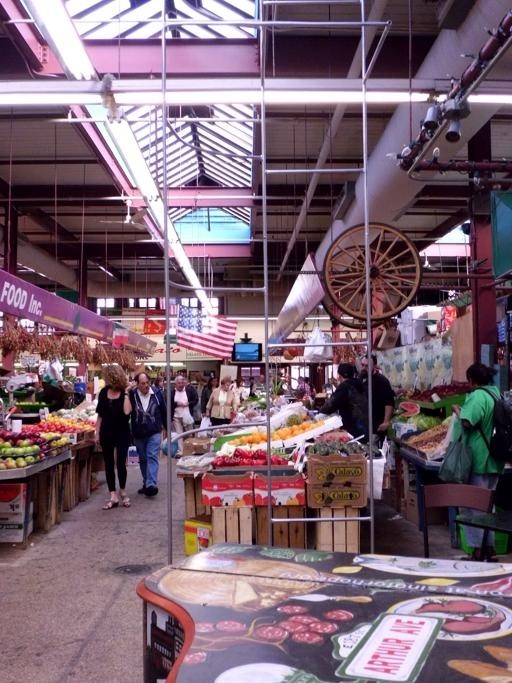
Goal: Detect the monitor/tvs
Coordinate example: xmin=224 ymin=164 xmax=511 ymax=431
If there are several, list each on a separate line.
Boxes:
xmin=232 ymin=343 xmax=262 ymax=361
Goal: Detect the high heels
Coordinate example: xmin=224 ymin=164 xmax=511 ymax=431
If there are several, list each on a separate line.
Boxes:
xmin=102 ymin=500 xmax=119 ymax=509
xmin=121 ymin=496 xmax=130 ymax=507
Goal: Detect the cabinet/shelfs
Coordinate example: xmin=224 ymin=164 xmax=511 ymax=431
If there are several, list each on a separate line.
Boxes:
xmin=387 ymin=392 xmax=470 ymax=470
xmin=0 ymin=404 xmax=96 ymax=480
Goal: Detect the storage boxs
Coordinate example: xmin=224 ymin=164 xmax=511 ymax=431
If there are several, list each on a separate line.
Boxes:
xmin=201 ymin=472 xmax=254 ymax=507
xmin=303 ymin=452 xmax=369 ymax=484
xmin=406 ymin=490 xmax=447 ymax=527
xmin=183 ymin=513 xmax=213 ymax=557
xmin=307 ymin=485 xmax=368 ymax=510
xmin=423 ymin=311 xmax=442 ymax=320
xmin=0 ymin=501 xmax=34 ymax=543
xmin=0 ymin=483 xmax=27 ymax=513
xmin=253 ymin=472 xmax=307 ymax=506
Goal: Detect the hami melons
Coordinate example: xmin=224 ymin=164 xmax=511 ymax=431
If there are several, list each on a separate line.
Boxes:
xmin=287 ymin=413 xmax=301 ymax=426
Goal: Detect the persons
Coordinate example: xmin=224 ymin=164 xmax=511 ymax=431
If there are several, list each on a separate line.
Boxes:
xmin=317 ymin=362 xmax=370 ymax=447
xmin=129 ymin=371 xmax=168 ymax=496
xmin=357 ymin=353 xmax=395 ymax=505
xmin=451 ymin=361 xmax=501 ymax=561
xmin=94 ymin=363 xmax=135 ymax=510
xmin=149 ymin=370 xmax=318 ymax=459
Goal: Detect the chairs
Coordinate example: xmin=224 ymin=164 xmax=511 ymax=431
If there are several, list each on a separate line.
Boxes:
xmin=420 ymin=482 xmax=496 ymax=561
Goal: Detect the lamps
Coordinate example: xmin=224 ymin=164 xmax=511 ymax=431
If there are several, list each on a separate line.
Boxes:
xmin=384 ymin=96 xmax=472 ymax=168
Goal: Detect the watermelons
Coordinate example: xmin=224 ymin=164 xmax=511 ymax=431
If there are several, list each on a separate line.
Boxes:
xmin=387 ymin=401 xmax=442 ymax=440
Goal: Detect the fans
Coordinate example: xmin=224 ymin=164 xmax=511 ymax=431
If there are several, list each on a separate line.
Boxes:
xmin=423 ymin=251 xmax=441 ymax=271
xmin=98 ymin=199 xmax=150 ymax=232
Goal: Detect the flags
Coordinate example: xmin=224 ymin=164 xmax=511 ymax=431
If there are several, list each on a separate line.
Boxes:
xmin=177 ymin=302 xmax=239 ymax=361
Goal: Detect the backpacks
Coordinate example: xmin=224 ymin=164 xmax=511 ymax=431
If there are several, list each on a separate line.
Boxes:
xmin=476 ymin=388 xmax=512 ymax=462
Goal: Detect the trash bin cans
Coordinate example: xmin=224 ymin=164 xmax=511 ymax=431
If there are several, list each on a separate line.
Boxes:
xmin=365 ymin=448 xmax=387 ymax=500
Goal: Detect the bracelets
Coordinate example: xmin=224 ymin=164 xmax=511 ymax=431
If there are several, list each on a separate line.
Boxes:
xmin=123 ymin=390 xmax=130 ymax=397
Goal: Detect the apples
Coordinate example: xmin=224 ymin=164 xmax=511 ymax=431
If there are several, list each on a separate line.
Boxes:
xmin=303 ymin=415 xmax=310 ymax=421
xmin=234 ymin=494 xmax=254 ymax=506
xmin=1 ymin=414 xmax=96 ymax=469
xmin=212 ymin=448 xmax=288 ymax=466
xmin=0 ymin=484 xmax=20 ymax=502
xmin=411 ymin=382 xmax=476 ymax=399
xmin=255 ymin=495 xmax=276 ymax=506
xmin=287 ymin=493 xmax=305 ymax=505
xmin=201 ymin=496 xmax=223 ymax=506
xmin=318 ymin=431 xmax=353 ymax=443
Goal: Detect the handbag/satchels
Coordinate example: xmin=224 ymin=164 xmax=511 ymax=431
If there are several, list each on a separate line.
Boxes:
xmin=438 ymin=441 xmax=472 ymax=483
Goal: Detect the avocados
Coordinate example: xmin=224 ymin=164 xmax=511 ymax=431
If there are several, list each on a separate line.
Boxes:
xmin=310 ymin=442 xmax=381 ymax=458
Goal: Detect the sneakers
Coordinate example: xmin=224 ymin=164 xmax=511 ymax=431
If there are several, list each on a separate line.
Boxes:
xmin=138 ymin=486 xmax=159 ymax=497
